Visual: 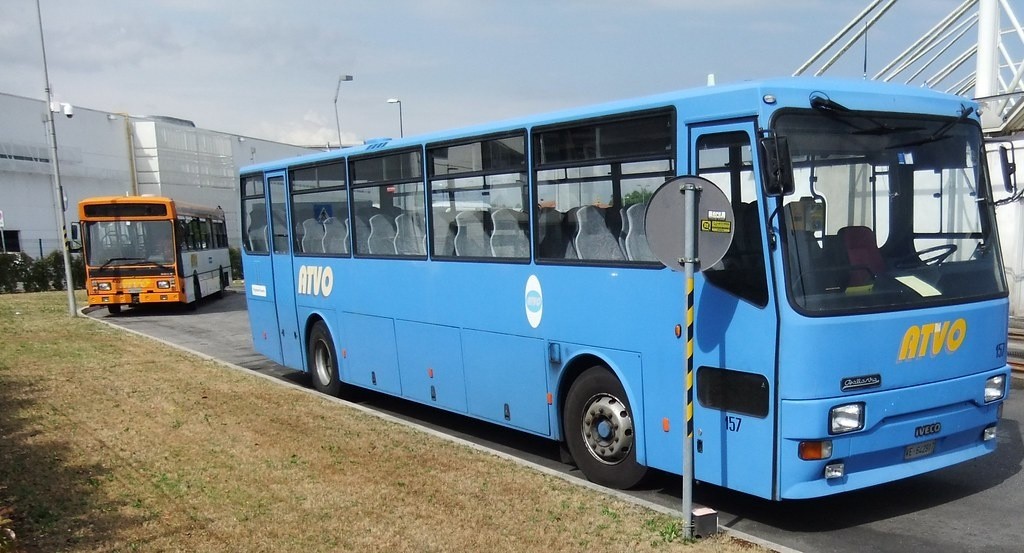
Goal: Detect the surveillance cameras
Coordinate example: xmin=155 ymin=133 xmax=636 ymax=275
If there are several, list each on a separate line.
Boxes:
xmin=64 ymin=105 xmax=73 ymax=118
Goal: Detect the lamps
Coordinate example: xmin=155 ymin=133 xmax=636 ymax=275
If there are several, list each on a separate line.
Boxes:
xmin=107 ymin=115 xmax=117 ymax=120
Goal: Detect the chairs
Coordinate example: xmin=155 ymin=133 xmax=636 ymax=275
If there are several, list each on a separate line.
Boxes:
xmin=247 ymin=203 xmax=656 ymax=263
xmin=836 ymin=226 xmax=886 ymax=286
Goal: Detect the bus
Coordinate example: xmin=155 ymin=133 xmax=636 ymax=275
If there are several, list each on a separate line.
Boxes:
xmin=237 ymin=78 xmax=1017 ymax=502
xmin=70 ymin=193 xmax=234 ymax=315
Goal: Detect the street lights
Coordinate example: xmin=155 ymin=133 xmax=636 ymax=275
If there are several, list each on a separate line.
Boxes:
xmin=386 ymin=98 xmax=403 ymax=139
xmin=334 ymin=75 xmax=354 ymax=149
